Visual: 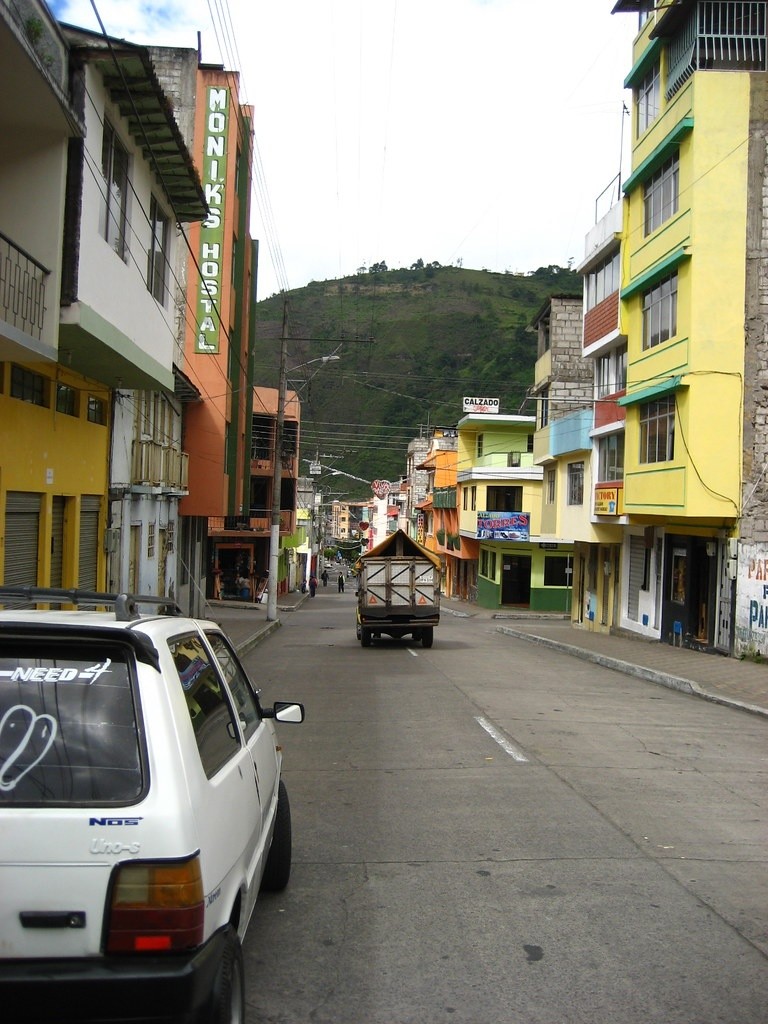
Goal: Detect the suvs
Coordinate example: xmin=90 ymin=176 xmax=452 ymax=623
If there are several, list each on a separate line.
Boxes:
xmin=1 ymin=585 xmax=305 ymax=1024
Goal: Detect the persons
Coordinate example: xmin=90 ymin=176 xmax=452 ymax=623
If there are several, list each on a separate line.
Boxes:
xmin=321 ymin=570 xmax=329 ymax=587
xmin=235 ymin=567 xmax=251 ymax=601
xmin=309 ymin=574 xmax=318 ymax=598
xmin=337 ymin=572 xmax=345 ymax=593
xmin=299 ymin=579 xmax=307 ymax=594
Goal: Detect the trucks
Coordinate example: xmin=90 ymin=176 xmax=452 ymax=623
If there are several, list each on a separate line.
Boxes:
xmin=355 ymin=536 xmax=441 ymax=648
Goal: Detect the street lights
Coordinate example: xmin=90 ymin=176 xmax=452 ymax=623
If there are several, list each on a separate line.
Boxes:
xmin=265 ymin=355 xmax=342 ymax=622
